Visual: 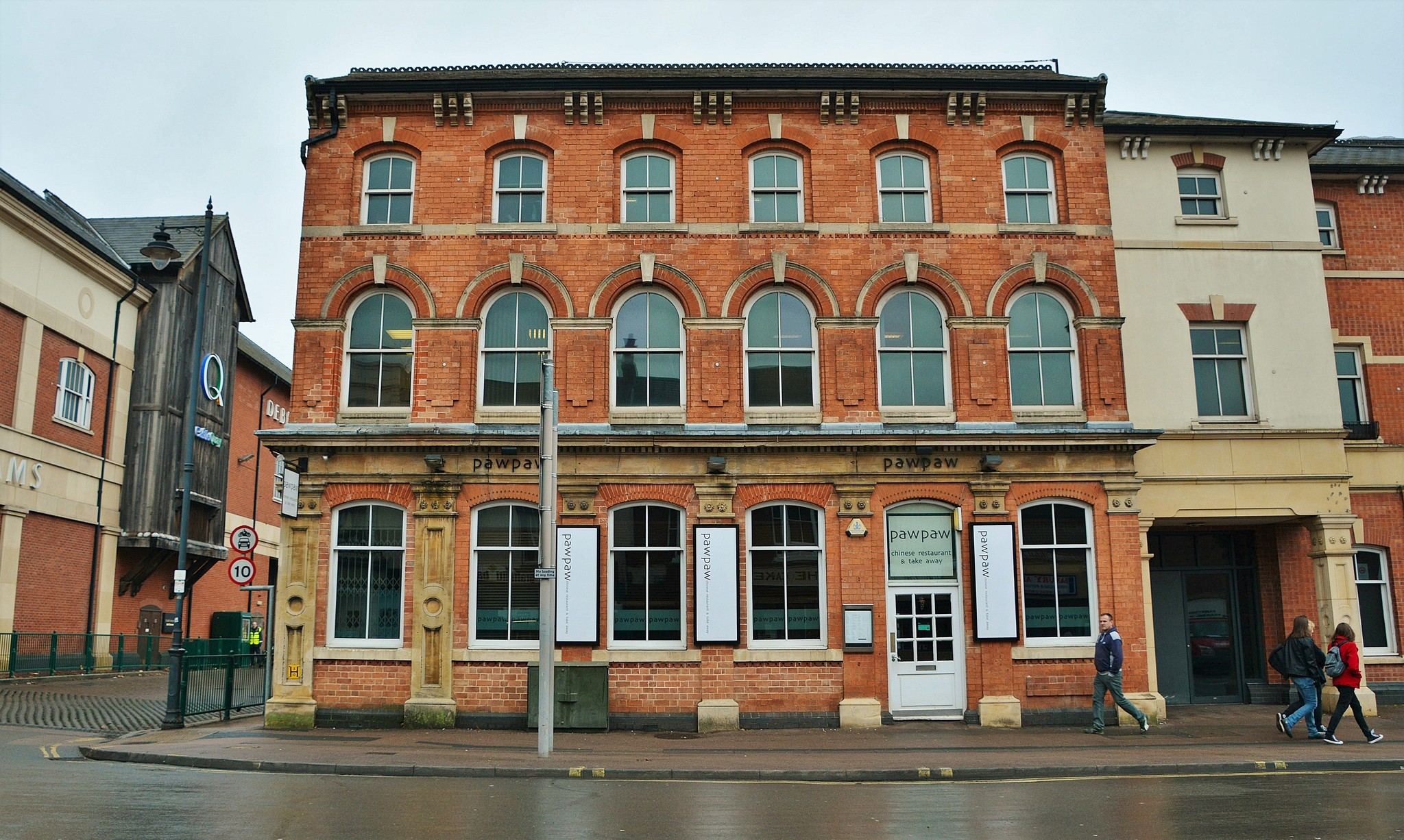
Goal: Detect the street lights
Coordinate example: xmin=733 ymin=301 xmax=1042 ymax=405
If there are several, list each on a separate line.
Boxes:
xmin=136 ymin=194 xmax=217 ymax=729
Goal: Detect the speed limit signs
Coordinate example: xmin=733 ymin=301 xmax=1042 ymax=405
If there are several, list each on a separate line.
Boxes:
xmin=228 ymin=557 xmax=257 ymax=585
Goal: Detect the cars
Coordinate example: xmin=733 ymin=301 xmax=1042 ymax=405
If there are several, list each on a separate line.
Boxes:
xmin=1188 ymin=617 xmax=1234 ymax=675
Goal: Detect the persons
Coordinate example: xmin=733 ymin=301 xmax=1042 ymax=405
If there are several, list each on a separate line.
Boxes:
xmin=249 ymin=622 xmax=264 ymax=670
xmin=1083 ymin=613 xmax=1152 ymax=735
xmin=1323 ymin=623 xmax=1384 ymax=745
xmin=1275 ymin=619 xmax=1328 ymax=736
xmin=1281 ymin=614 xmax=1324 ymax=741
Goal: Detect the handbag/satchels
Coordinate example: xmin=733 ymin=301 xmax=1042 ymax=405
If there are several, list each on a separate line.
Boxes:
xmin=1268 ymin=635 xmax=1291 ymax=680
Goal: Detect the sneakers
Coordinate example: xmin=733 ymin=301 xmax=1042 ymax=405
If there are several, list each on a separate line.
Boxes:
xmin=1318 ymin=724 xmax=1329 ymax=732
xmin=1276 ymin=712 xmax=1286 ymax=733
xmin=1083 ymin=726 xmax=1105 ymax=734
xmin=1308 ymin=733 xmax=1324 ymax=739
xmin=1280 ymin=718 xmax=1294 ymax=739
xmin=1368 ymin=733 xmax=1384 ymax=743
xmin=1138 ymin=713 xmax=1150 ymax=733
xmin=1324 ymin=734 xmax=1344 ymax=744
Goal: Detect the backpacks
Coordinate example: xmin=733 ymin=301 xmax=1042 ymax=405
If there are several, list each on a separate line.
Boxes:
xmin=1324 ymin=639 xmax=1352 ymax=677
